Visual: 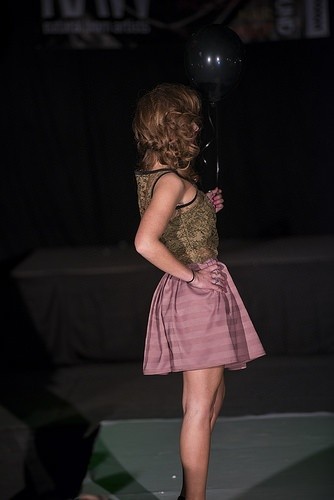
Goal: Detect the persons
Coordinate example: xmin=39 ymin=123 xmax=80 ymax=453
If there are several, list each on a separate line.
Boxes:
xmin=130 ymin=84 xmax=266 ymax=500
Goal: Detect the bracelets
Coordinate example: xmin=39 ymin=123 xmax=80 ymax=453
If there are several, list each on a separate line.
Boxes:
xmin=186 ymin=268 xmax=195 ymax=284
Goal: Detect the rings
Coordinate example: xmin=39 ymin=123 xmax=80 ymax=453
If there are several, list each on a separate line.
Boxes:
xmin=208 ymin=190 xmax=214 ymax=196
xmin=214 ymin=277 xmax=219 ymax=285
